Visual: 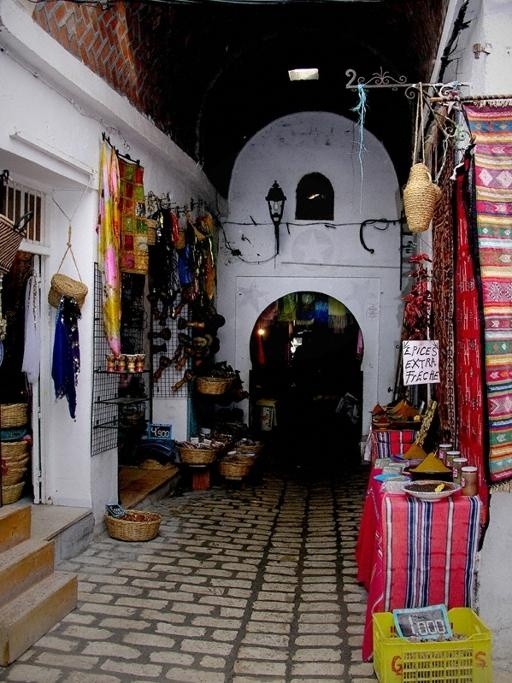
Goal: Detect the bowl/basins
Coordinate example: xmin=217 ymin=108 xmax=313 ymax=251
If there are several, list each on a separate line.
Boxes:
xmin=405 ymin=466 xmax=452 ymax=481
xmin=400 ymin=479 xmax=462 ymax=503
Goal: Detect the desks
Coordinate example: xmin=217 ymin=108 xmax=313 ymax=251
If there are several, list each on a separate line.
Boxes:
xmin=357 ymin=456 xmax=477 ymax=661
xmin=365 ymin=415 xmax=417 ymax=467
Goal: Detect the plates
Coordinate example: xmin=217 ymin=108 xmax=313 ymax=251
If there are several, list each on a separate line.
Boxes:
xmin=390 ymin=452 xmax=425 ymax=463
xmin=369 ymin=408 xmax=414 ymax=429
xmin=373 ymin=474 xmax=412 ymax=483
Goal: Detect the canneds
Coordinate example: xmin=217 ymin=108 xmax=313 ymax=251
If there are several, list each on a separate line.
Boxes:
xmin=439 ymin=443 xmax=478 ymax=496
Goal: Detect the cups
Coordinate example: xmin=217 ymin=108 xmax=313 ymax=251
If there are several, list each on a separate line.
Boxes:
xmin=438 ymin=444 xmax=453 ymax=461
xmin=446 ymin=450 xmax=461 ymax=470
xmin=382 ymin=466 xmax=401 ymax=478
xmin=460 ymin=466 xmax=477 ymax=496
xmin=452 ymin=458 xmax=469 ymax=485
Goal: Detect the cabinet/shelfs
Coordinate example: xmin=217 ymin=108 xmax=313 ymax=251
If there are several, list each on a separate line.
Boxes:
xmin=93 ymin=367 xmax=150 ymax=434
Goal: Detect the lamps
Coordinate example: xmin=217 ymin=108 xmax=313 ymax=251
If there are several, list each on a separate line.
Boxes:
xmin=266 ymin=178 xmax=287 ymax=231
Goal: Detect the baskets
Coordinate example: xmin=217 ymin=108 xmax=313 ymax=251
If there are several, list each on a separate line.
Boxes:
xmin=46 ymin=272 xmax=90 ymax=313
xmin=219 ymin=438 xmax=266 ymax=478
xmin=370 ymin=605 xmax=494 ymax=683
xmin=103 ymin=507 xmax=164 ymax=542
xmin=178 ymin=429 xmax=237 ymax=464
xmin=0 ymin=401 xmax=31 ymax=505
xmin=195 ymin=374 xmax=230 ymax=395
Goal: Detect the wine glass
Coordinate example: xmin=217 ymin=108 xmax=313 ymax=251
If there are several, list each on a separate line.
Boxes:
xmin=105 ymin=353 xmax=145 ymax=373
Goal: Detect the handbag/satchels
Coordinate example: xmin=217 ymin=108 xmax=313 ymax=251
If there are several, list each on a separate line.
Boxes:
xmin=0 ymin=209 xmax=35 ymax=275
